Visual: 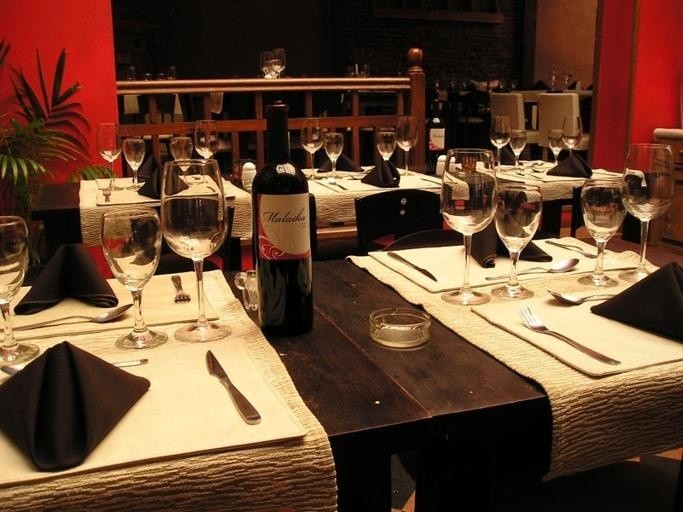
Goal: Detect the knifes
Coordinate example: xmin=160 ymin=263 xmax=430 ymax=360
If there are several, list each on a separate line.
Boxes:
xmin=202 ymin=349 xmax=262 ymax=428
xmin=386 ymin=252 xmax=439 ymax=283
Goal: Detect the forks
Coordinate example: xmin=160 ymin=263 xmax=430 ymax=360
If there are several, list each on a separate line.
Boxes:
xmin=171 ymin=274 xmax=192 ymax=304
xmin=518 ymin=303 xmax=623 ymax=366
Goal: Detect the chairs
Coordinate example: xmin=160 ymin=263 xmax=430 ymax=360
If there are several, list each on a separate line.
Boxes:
xmin=489 ymin=92 xmax=539 ymax=161
xmin=538 ymin=92 xmax=591 ymax=162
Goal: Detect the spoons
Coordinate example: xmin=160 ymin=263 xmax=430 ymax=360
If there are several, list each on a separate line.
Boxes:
xmin=484 ymin=256 xmax=580 ymax=281
xmin=545 ymin=240 xmax=608 ymax=259
xmin=9 ymin=302 xmax=134 ymax=332
xmin=1 ymin=358 xmax=148 ymax=382
xmin=546 ymin=288 xmax=621 ymax=304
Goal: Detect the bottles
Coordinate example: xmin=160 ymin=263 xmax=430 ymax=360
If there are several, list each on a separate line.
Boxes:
xmin=250 ymin=97 xmax=315 ymax=339
xmin=425 ymin=97 xmax=448 ymax=177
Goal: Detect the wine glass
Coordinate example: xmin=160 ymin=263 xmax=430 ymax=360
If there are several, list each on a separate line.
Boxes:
xmin=121 ymin=137 xmax=146 ymax=190
xmin=620 ymin=142 xmax=677 ymax=285
xmin=375 ymin=132 xmax=398 ymax=161
xmin=397 ymin=114 xmax=420 ymax=176
xmin=549 ymin=127 xmax=565 ymax=166
xmin=563 ymin=116 xmax=583 ymax=150
xmin=491 ymin=183 xmax=543 ymax=302
xmin=300 ymin=119 xmax=325 ymax=180
xmin=0 ymin=213 xmax=44 ymax=366
xmin=161 ymin=158 xmax=232 ymax=344
xmin=577 ymin=176 xmax=628 ymax=288
xmin=97 ymin=121 xmax=126 ymax=192
xmin=194 ymin=119 xmax=218 ymax=184
xmin=489 ymin=115 xmax=511 ymax=173
xmin=169 ymin=137 xmax=194 ymax=187
xmin=99 ymin=208 xmax=168 ymax=351
xmin=509 ymin=130 xmax=526 ymax=171
xmin=440 ymin=147 xmax=497 ymax=307
xmin=321 ymin=131 xmax=344 ymax=179
